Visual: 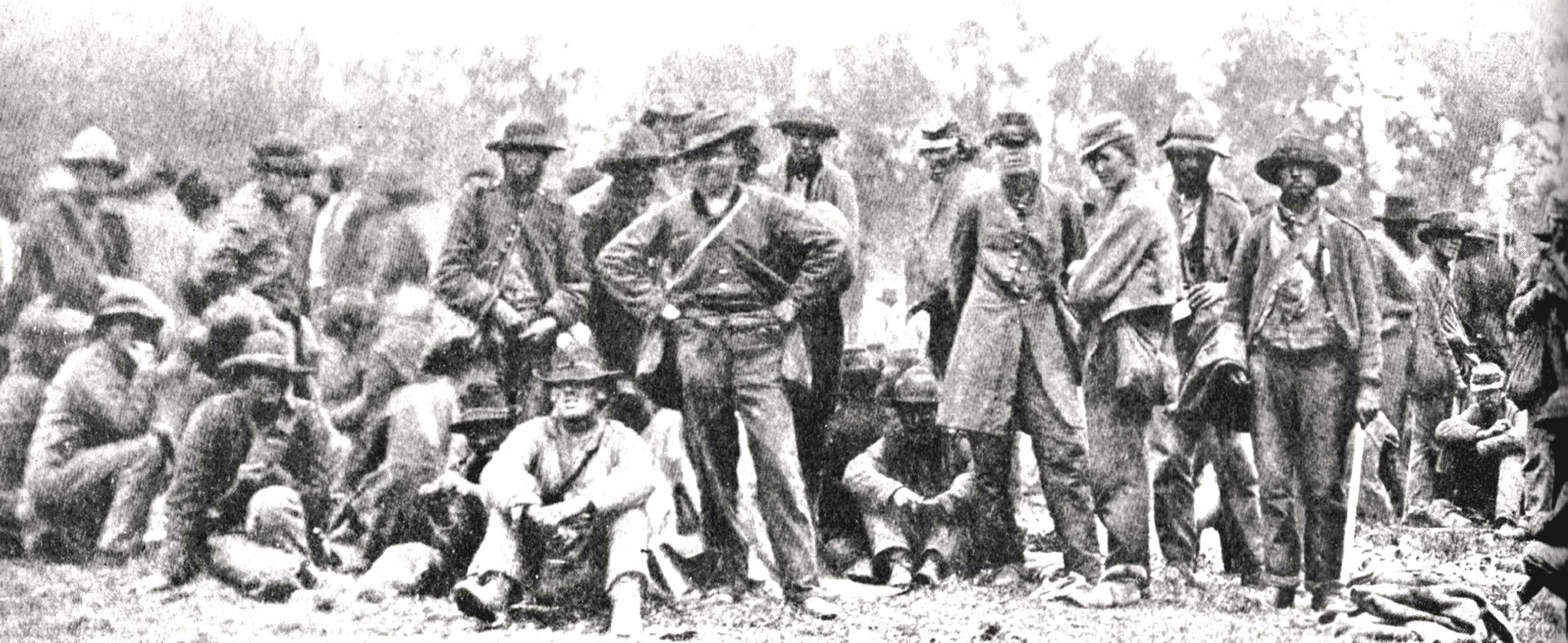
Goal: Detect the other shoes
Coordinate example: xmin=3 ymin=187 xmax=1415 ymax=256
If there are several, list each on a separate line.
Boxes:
xmin=452 ymin=559 xmax=1357 ymax=625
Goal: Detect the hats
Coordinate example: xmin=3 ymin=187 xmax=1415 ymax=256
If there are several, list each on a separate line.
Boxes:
xmin=983 ymin=112 xmax=1040 ymax=147
xmin=594 ymin=127 xmax=666 ymax=169
xmin=1372 ymin=195 xmax=1431 ymax=224
xmin=1462 ymin=219 xmax=1495 ymax=243
xmin=57 ymin=127 xmax=123 ymax=176
xmin=217 ymin=330 xmax=316 ymax=376
xmin=484 ymin=121 xmax=565 ymax=154
xmin=1078 ymin=118 xmax=1132 ymax=163
xmin=916 ymin=109 xmax=963 ymax=150
xmin=245 ymin=133 xmax=313 ymax=177
xmin=675 ymin=109 xmax=754 ymax=157
xmin=1254 ymin=131 xmax=1340 ymax=187
xmin=448 ymin=380 xmax=523 ymax=435
xmin=878 ymin=366 xmax=941 ymax=406
xmin=1469 ymin=363 xmax=1503 ymax=392
xmin=531 ymin=343 xmax=623 ymax=382
xmin=93 ymin=292 xmax=163 ymax=335
xmin=1155 ymin=109 xmax=1231 ymax=159
xmin=1418 ymin=210 xmax=1465 ymax=243
xmin=770 ymin=103 xmax=838 ymax=140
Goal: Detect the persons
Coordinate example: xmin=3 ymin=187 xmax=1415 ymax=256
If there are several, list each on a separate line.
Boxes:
xmin=1216 ymin=127 xmax=1383 ymax=616
xmin=594 ymin=106 xmax=849 ymax=621
xmin=0 ymin=104 xmax=1568 ymax=639
xmin=1063 ymin=111 xmax=1180 ymax=607
xmin=454 ymin=342 xmax=658 ymax=639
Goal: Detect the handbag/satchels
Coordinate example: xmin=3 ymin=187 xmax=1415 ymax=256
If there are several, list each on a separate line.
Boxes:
xmin=1505 ymin=320 xmax=1555 ymax=400
xmin=1114 ymin=327 xmax=1177 ymax=407
